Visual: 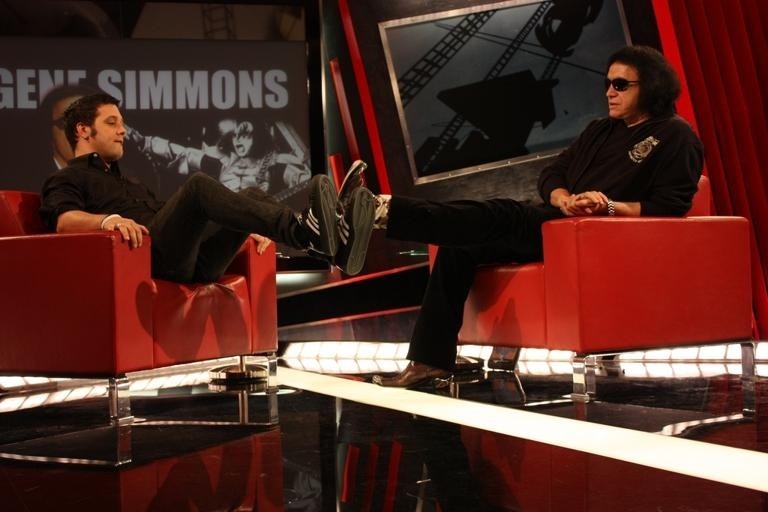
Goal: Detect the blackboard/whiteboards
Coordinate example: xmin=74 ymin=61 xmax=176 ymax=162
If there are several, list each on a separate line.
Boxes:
xmin=376 ymin=0 xmax=638 ymax=188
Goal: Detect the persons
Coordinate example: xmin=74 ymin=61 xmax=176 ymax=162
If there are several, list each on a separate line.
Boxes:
xmin=338 ymin=43 xmax=705 ymax=387
xmin=41 ymin=91 xmax=375 ymax=285
xmin=36 ymin=86 xmax=182 ymax=202
xmin=173 ymin=115 xmax=311 ymax=194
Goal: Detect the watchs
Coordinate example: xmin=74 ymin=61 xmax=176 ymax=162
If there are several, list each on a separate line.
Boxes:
xmin=608 ymin=198 xmax=615 ymax=216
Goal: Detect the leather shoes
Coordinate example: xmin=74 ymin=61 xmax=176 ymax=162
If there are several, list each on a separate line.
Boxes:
xmin=334 ymin=159 xmax=368 ymax=214
xmin=373 ymin=359 xmax=453 ymax=390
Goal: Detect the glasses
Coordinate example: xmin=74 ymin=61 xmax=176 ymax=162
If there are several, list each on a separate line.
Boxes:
xmin=604 ymin=77 xmax=642 ymax=92
xmin=49 ymin=115 xmax=67 ymax=131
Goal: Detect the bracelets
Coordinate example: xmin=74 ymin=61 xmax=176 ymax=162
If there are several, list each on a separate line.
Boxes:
xmin=100 ymin=212 xmax=121 ymax=231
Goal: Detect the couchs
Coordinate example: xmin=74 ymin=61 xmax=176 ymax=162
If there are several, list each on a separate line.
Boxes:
xmin=0 ymin=190 xmax=278 ymax=470
xmin=1 ymin=424 xmax=287 ymax=512
xmin=459 ymin=375 xmax=767 ymax=511
xmin=428 ymin=175 xmax=755 ymax=403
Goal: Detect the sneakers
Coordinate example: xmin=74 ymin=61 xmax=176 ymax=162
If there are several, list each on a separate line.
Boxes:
xmin=295 ymin=174 xmax=339 ymax=259
xmin=332 ymin=187 xmax=377 ymax=278
